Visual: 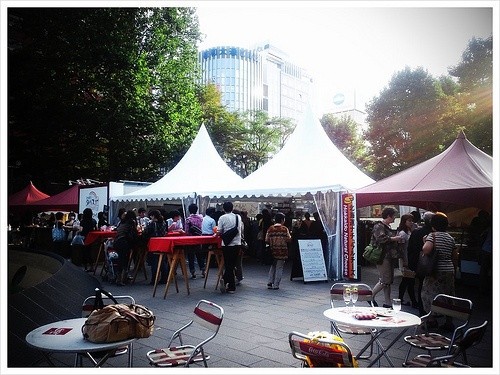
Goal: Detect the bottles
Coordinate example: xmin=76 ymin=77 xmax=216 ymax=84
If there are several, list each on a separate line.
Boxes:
xmin=142 ymin=225 xmax=144 ymax=231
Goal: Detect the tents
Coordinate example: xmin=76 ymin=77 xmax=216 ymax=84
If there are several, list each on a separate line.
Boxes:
xmin=109 ymin=121 xmax=243 ymax=235
xmin=7 ymin=179 xmax=84 ymax=246
xmin=243 ymin=100 xmax=376 ymax=282
xmin=349 ymin=129 xmax=493 ymax=282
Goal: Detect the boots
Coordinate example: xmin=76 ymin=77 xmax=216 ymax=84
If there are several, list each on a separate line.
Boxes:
xmin=150 ymin=268 xmax=168 ymax=284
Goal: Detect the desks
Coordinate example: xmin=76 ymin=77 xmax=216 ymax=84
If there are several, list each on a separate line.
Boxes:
xmin=84 ymin=231 xmax=117 ymax=277
xmin=323 ymin=306 xmax=422 ymax=367
xmin=148 ymin=236 xmax=227 ymax=300
xmin=25 ymin=317 xmax=137 ymax=368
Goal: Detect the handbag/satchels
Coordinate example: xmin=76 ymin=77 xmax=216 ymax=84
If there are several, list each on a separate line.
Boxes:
xmin=71 ymin=235 xmax=84 ymax=246
xmin=415 ymin=254 xmax=434 ymax=276
xmin=52 ymin=221 xmax=66 ymax=241
xmin=362 ymin=245 xmax=384 ymax=265
xmin=220 ymin=227 xmax=239 ymax=246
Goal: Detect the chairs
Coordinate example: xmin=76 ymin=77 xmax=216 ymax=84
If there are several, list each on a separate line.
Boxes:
xmin=402 ymin=319 xmax=487 ymax=368
xmin=403 ymin=293 xmax=473 ymax=368
xmin=330 ymin=282 xmax=375 ymax=360
xmin=73 ymin=295 xmax=136 ymax=368
xmin=288 ymin=331 xmax=355 ymax=368
xmin=146 ymin=299 xmax=224 ymax=368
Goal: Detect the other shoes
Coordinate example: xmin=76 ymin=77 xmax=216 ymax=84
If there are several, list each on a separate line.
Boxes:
xmin=202 ymin=271 xmax=206 ymax=278
xmin=266 ymin=283 xmax=272 ymax=288
xmin=190 ymin=275 xmax=196 ymax=280
xmin=368 ymin=300 xmax=378 ymax=307
xmin=401 ymin=300 xmax=410 ymax=305
xmin=84 ymin=268 xmax=134 ymax=286
xmin=226 ymin=288 xmax=235 ymax=294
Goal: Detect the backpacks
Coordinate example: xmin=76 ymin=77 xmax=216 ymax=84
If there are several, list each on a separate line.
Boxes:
xmin=82 ymin=303 xmax=154 ymax=342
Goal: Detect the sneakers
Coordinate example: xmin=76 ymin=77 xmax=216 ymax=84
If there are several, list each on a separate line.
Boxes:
xmin=422 ymin=321 xmax=454 ymax=333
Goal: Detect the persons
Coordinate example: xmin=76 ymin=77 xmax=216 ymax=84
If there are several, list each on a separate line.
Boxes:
xmin=115 ymin=203 xmax=250 ymax=284
xmin=366 ymin=206 xmax=493 ymax=339
xmin=7 ymin=208 xmax=114 ymax=282
xmin=216 ymin=201 xmax=244 ymax=294
xmin=256 ymin=209 xmax=328 ymax=269
xmin=265 ymin=212 xmax=292 ymax=289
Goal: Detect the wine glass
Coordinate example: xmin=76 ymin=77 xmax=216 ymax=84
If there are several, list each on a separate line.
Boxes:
xmin=392 ymin=299 xmax=401 ymax=313
xmin=350 ymin=284 xmax=358 ymax=311
xmin=342 ymin=284 xmax=352 ymax=313
